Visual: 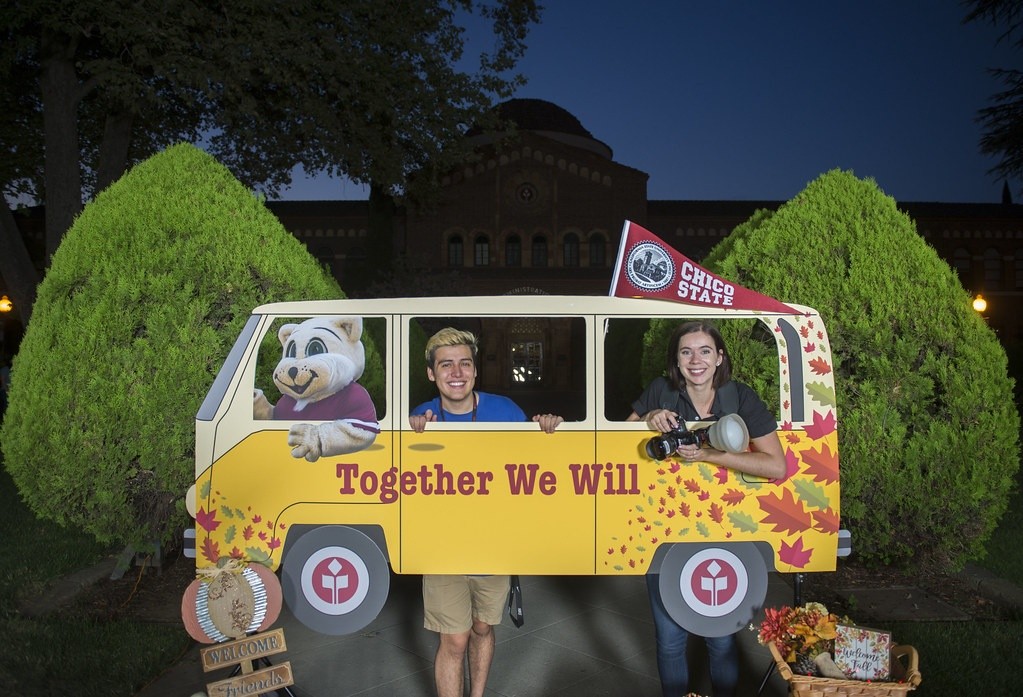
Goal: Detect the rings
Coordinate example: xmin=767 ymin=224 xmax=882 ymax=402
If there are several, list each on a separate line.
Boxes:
xmin=694 ymin=450 xmax=696 ymax=455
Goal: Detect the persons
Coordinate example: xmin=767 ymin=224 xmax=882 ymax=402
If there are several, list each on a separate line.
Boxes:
xmin=410 ymin=327 xmax=564 ymax=697
xmin=624 ymin=319 xmax=787 ymax=697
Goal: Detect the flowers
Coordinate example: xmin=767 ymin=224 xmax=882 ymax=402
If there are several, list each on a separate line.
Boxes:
xmin=749 ymin=600 xmax=856 ymax=664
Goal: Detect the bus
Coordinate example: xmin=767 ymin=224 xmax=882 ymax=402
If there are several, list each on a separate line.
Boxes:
xmin=183 ymin=295 xmax=852 ymax=637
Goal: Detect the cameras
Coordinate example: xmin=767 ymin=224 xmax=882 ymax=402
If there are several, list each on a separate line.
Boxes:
xmin=646 ymin=413 xmax=749 ymax=461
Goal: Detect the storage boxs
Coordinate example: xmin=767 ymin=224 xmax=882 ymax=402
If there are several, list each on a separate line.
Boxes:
xmin=833 ymin=622 xmax=890 ymax=682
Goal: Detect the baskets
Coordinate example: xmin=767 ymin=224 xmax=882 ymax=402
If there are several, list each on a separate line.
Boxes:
xmin=767 ymin=622 xmax=923 ymax=697
